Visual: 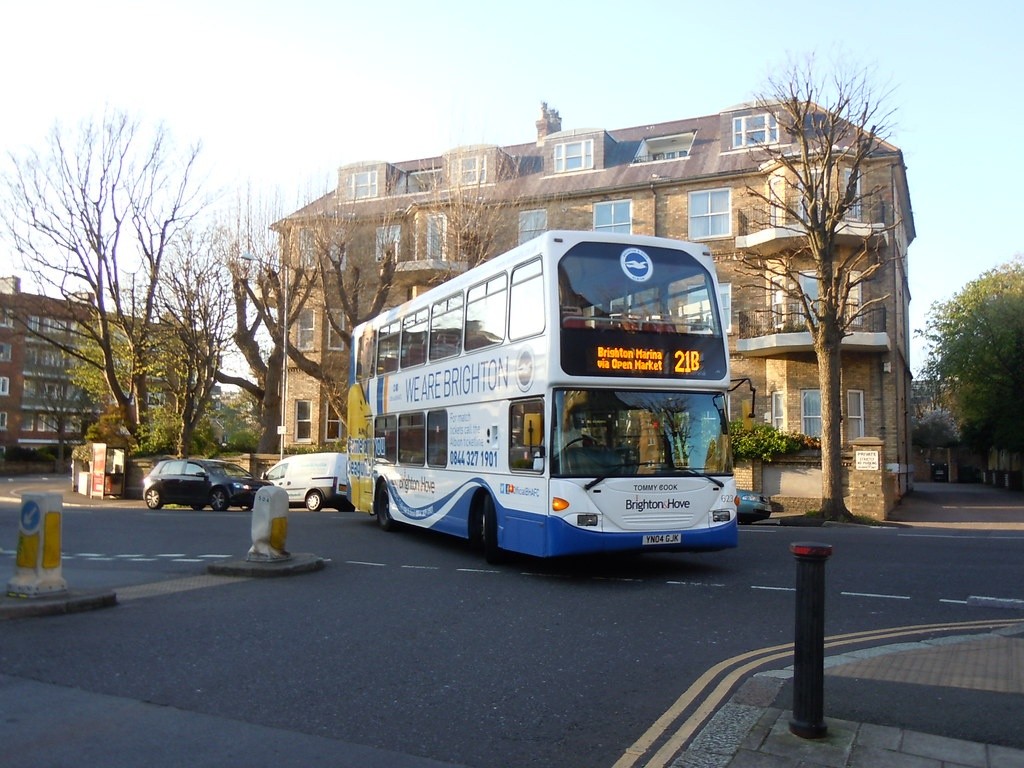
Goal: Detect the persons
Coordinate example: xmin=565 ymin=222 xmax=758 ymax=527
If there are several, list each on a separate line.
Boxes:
xmin=540 ymin=410 xmax=583 ymax=458
xmin=347 ymin=323 xmax=376 ymax=513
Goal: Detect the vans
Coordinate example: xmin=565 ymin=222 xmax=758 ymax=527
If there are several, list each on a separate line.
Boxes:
xmin=258 ymin=452 xmax=356 ymax=512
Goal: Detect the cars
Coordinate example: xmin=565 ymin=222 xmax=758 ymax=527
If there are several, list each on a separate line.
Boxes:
xmin=736 ymin=489 xmax=772 ymax=525
xmin=142 ymin=459 xmax=274 ymax=512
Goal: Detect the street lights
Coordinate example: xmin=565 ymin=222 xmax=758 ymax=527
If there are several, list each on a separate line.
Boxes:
xmin=240 ymin=252 xmax=288 ymax=463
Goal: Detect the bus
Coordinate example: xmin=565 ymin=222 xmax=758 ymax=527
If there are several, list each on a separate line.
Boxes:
xmin=345 ymin=229 xmax=757 ymax=566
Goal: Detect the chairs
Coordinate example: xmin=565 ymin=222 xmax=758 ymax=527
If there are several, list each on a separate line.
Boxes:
xmin=377 ymin=426 xmax=447 ymax=466
xmin=560 ymin=305 xmax=676 ymax=331
